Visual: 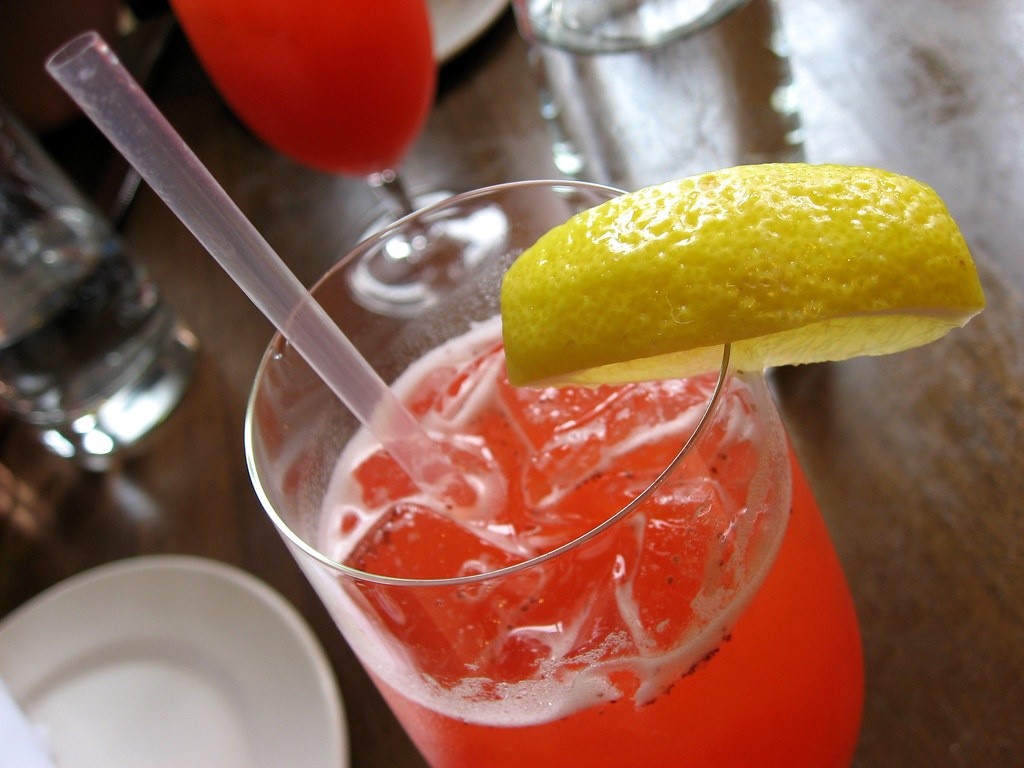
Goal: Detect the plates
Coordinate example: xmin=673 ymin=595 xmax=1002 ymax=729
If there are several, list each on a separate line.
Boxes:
xmin=0 ymin=550 xmax=350 ymax=768
xmin=426 ymin=0 xmax=509 ymax=63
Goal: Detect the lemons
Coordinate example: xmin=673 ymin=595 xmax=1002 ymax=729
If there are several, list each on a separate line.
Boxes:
xmin=501 ymin=163 xmax=987 ymax=387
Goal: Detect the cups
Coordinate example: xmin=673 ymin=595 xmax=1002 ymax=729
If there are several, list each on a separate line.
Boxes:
xmin=242 ymin=178 xmax=865 ymax=768
xmin=525 ymin=0 xmax=806 ymax=193
xmin=0 ymin=104 xmax=195 ymax=475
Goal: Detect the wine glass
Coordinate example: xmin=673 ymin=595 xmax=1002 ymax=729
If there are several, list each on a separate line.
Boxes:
xmin=170 ymin=0 xmax=510 ymax=321
